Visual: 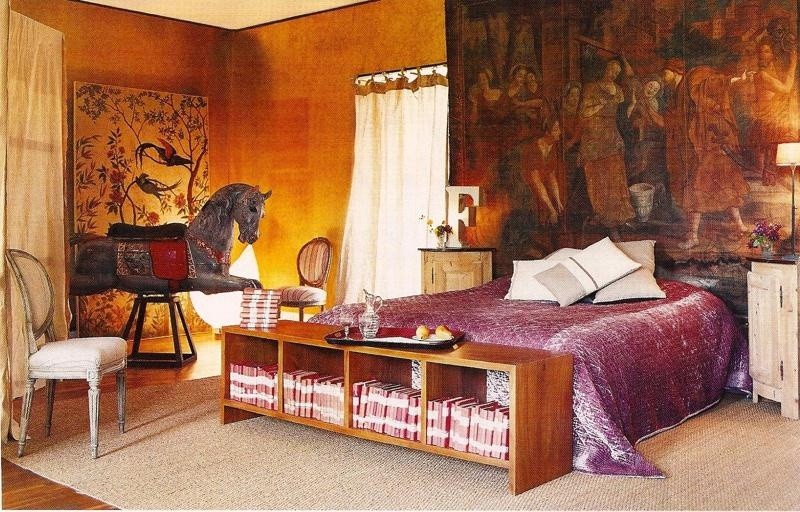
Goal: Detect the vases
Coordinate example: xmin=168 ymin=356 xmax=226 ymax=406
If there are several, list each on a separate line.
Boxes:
xmin=435 ymin=231 xmax=449 ymax=248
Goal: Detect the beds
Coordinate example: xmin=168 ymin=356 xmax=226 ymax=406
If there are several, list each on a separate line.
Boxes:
xmin=305 ymin=277 xmax=750 ymax=478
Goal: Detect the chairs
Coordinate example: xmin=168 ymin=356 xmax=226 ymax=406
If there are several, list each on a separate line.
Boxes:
xmin=274 ymin=234 xmax=332 ymax=323
xmin=3 ymin=247 xmax=130 ymax=460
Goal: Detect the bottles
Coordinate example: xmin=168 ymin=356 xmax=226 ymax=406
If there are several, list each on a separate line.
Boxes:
xmin=358 ymin=289 xmax=383 ymax=339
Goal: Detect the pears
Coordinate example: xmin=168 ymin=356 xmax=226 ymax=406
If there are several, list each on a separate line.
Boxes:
xmin=434 ymin=326 xmax=453 ymax=339
xmin=415 ymin=326 xmax=430 ymax=339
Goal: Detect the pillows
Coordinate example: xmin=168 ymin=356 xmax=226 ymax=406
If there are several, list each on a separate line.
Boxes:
xmin=593 ymin=239 xmax=668 ymax=305
xmin=541 ymin=247 xmax=581 ymax=263
xmin=534 ymin=236 xmax=642 ymax=309
xmin=505 ymin=260 xmax=558 ymax=303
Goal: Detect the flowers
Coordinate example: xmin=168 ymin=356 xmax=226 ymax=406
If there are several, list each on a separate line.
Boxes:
xmin=418 ymin=214 xmax=453 ymax=235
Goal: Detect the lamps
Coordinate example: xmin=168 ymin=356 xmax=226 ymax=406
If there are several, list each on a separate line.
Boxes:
xmin=776 ymin=141 xmax=800 ymax=256
xmin=188 ymin=243 xmax=260 ymax=338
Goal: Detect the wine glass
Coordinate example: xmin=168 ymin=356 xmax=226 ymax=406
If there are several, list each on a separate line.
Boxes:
xmin=339 ymin=303 xmax=354 ymax=341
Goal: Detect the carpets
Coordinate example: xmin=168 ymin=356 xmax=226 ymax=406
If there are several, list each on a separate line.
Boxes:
xmin=2 ymin=374 xmax=800 ymax=510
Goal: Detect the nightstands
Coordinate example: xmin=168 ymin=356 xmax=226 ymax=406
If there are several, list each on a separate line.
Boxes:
xmin=746 ymin=253 xmax=800 ymax=419
xmin=416 ymin=245 xmax=496 ymax=294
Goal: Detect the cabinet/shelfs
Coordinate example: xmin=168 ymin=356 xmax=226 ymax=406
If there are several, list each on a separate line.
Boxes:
xmin=219 ymin=317 xmax=575 ymax=497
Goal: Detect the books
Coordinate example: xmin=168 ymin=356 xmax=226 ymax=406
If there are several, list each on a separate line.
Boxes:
xmin=239 ymin=287 xmax=282 ymax=328
xmin=229 ymin=357 xmax=510 ymax=464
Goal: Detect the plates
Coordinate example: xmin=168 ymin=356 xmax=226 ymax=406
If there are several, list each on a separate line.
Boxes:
xmin=412 ymin=334 xmax=454 ymax=341
